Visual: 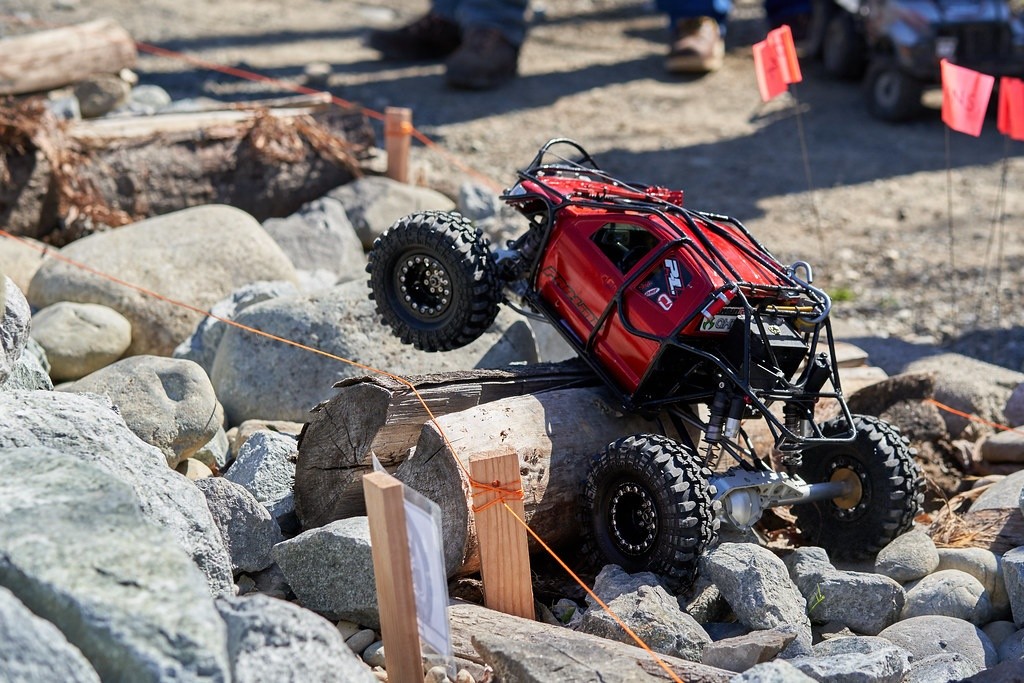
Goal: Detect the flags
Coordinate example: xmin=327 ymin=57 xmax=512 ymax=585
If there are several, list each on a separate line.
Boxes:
xmin=999 ymin=78 xmax=1024 ymax=140
xmin=752 ymin=30 xmax=801 ymax=101
xmin=941 ymin=59 xmax=994 ymax=137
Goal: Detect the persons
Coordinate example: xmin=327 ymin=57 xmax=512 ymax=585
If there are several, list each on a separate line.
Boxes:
xmin=363 ymin=0 xmax=532 ymax=87
xmin=654 ymin=0 xmax=815 ymax=72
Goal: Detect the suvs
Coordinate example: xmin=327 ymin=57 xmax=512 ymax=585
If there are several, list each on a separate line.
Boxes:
xmin=365 ymin=137 xmax=931 ymax=589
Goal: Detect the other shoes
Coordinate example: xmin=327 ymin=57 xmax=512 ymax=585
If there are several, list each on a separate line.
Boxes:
xmin=662 ymin=14 xmax=725 ymax=73
xmin=436 ymin=25 xmax=519 ymax=90
xmin=360 ymin=8 xmax=463 ymax=62
